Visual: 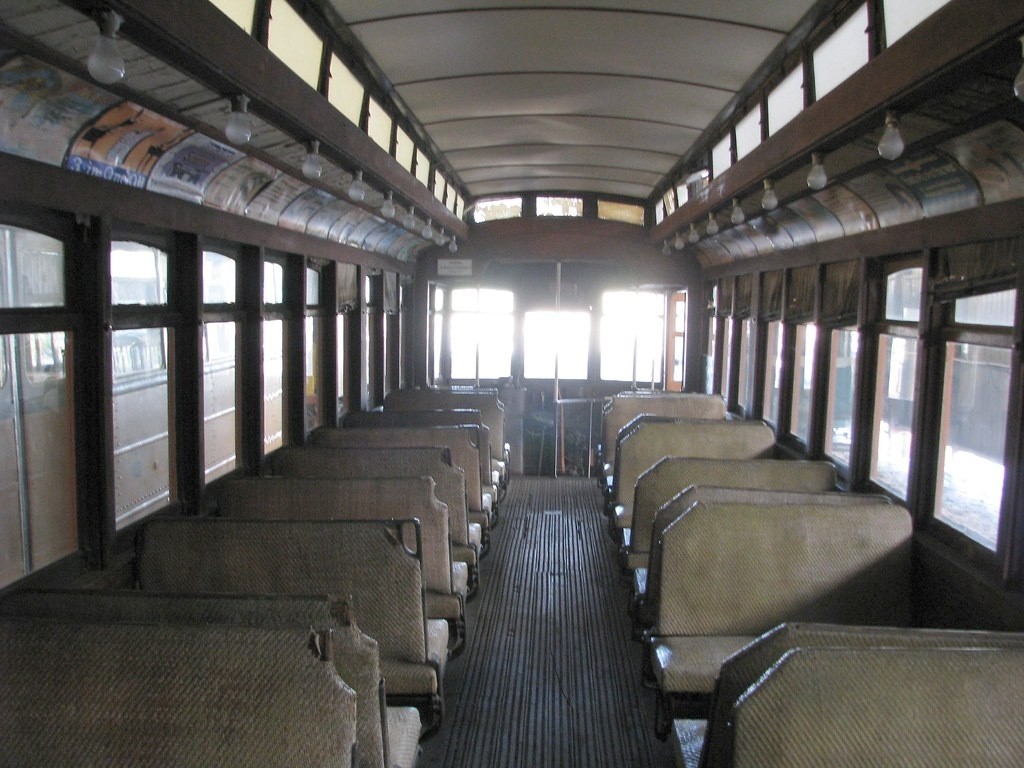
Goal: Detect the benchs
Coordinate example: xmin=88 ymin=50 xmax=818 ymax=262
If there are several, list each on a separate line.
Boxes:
xmin=0 ymin=386 xmax=512 ymax=768
xmin=599 ymin=389 xmax=1024 ymax=768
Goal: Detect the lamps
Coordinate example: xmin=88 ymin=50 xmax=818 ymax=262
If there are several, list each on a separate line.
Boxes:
xmin=663 ymin=239 xmax=671 ymax=256
xmin=707 ymin=212 xmax=718 ymax=233
xmin=807 ymin=152 xmax=827 ymax=190
xmin=88 ymin=11 xmax=124 ymax=84
xmin=436 ymin=227 xmax=444 ymax=246
xmin=224 ymin=95 xmax=251 ymax=145
xmin=302 ymin=140 xmax=322 ymax=179
xmin=449 ymin=235 xmax=457 ymax=252
xmin=762 ymin=178 xmax=778 ymax=210
xmin=381 ymin=190 xmax=395 ymax=218
xmin=1013 ymin=36 xmax=1024 ymax=101
xmin=404 ymin=206 xmax=415 ymax=229
xmin=731 ymin=198 xmax=745 ymax=224
xmin=422 ymin=218 xmax=432 ymax=239
xmin=878 ymin=109 xmax=904 ymax=160
xmin=349 ymin=170 xmax=365 ymax=201
xmin=689 ymin=223 xmax=698 ymax=243
xmin=675 ymin=232 xmax=685 ymax=250
